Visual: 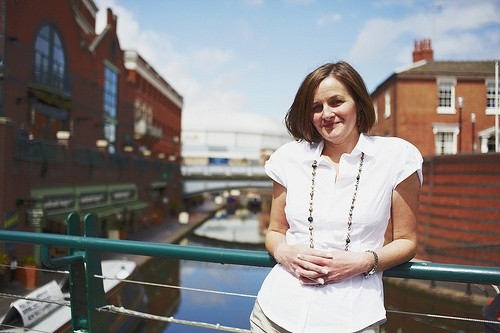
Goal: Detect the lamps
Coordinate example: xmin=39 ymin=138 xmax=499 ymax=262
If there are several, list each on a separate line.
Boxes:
xmin=96 ymin=138 xmax=110 ymax=148
xmin=123 ymin=142 xmax=134 ymax=151
xmin=141 ymin=148 xmax=151 ymax=156
xmin=55 ymin=130 xmax=71 ymax=140
xmin=157 ymin=151 xmax=184 ymax=162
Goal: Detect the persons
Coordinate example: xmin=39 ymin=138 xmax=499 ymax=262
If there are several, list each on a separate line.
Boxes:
xmin=250 ymin=60 xmax=425 ymax=333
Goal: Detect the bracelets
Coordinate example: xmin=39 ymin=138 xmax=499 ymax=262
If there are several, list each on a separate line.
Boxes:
xmin=361 ymin=250 xmax=378 ymax=278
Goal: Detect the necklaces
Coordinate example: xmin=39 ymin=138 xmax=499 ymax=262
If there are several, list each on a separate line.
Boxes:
xmin=308 ymin=153 xmax=365 ymax=251
